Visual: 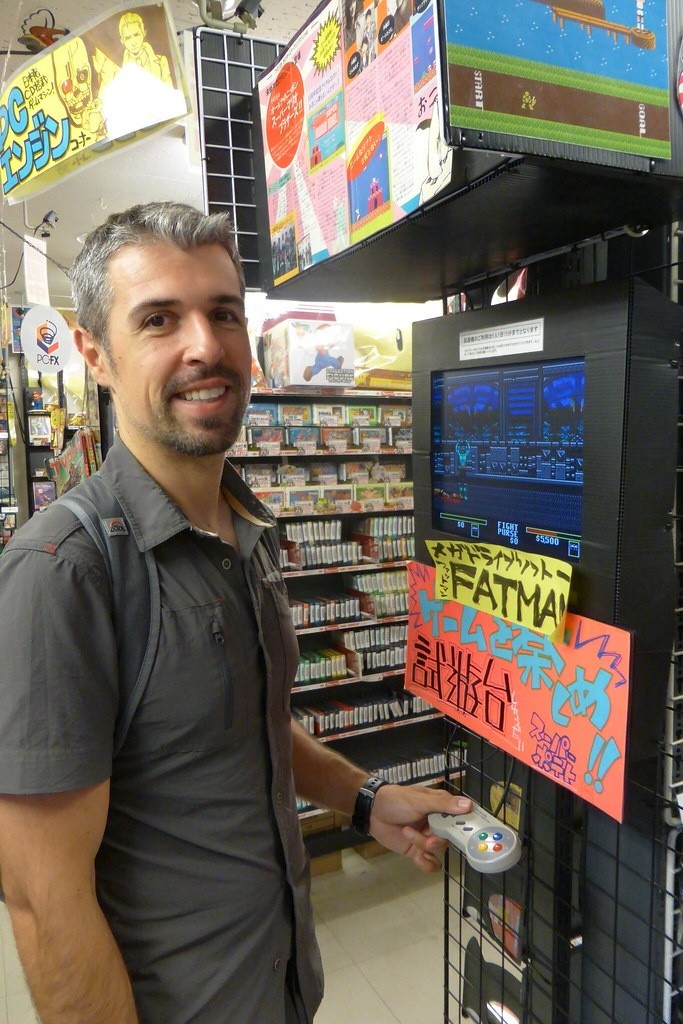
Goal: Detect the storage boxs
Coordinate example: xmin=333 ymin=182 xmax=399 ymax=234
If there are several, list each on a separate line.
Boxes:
xmin=263 ymin=318 xmax=355 ymax=387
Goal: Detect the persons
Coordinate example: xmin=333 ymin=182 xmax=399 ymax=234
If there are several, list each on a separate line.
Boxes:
xmin=0 ymin=201 xmax=473 ymax=1024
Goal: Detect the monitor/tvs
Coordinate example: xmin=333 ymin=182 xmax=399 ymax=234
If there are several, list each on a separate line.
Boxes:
xmin=431 ymin=355 xmax=586 ymax=566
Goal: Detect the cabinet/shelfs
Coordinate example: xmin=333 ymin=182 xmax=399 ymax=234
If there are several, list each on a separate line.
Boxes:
xmin=0 ymin=345 xmax=19 ymax=555
xmin=21 ymin=353 xmax=83 ymax=519
xmin=97 ymin=384 xmax=467 ymax=876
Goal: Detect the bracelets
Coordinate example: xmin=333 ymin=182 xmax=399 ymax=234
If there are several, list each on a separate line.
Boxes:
xmin=353 ymin=776 xmax=389 ymax=839
